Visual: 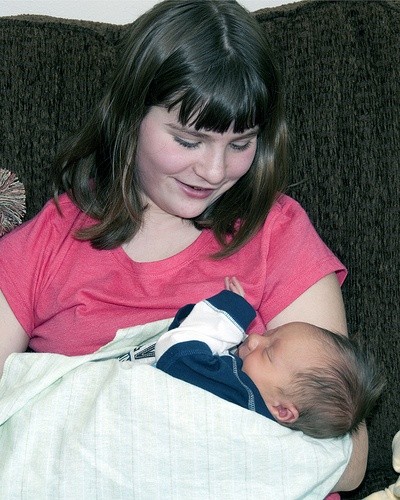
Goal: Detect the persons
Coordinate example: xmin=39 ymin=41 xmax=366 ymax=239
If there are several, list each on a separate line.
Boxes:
xmin=0 ymin=275 xmax=388 ymax=500
xmin=0 ymin=0 xmax=369 ymax=500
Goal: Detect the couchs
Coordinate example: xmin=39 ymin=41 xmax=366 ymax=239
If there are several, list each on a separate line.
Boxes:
xmin=1 ymin=0 xmax=400 ymax=500
